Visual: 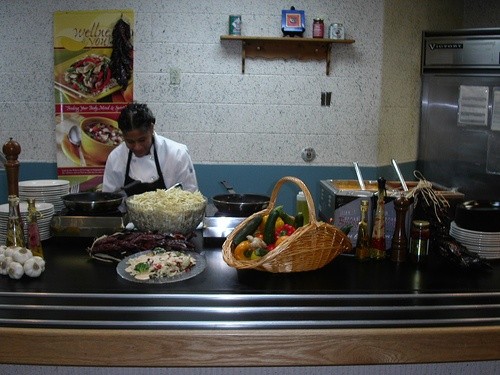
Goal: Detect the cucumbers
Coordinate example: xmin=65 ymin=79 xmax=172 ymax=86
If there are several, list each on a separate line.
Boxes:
xmin=264 ymin=206 xmax=283 ymax=243
xmin=294 ymin=212 xmax=303 ymax=228
xmin=233 ymin=216 xmax=262 ymax=246
xmin=340 ymin=225 xmax=350 ymax=235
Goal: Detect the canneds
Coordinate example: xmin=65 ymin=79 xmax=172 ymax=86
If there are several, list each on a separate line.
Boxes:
xmin=328 ymin=23 xmax=345 ymax=39
xmin=409 ymin=220 xmax=430 ymax=256
xmin=229 ymin=15 xmax=241 ymax=36
xmin=312 ymin=18 xmax=324 ymax=39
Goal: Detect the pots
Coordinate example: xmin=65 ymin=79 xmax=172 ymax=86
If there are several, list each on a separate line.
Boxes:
xmin=211 ymin=179 xmax=270 ymax=217
xmin=61 ymin=180 xmax=141 ymax=213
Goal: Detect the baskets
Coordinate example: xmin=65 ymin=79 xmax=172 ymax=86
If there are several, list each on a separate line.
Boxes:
xmin=222 ymin=176 xmax=352 ymax=274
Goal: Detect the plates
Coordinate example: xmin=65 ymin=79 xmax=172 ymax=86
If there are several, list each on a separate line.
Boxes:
xmin=61 ymin=133 xmax=107 ymax=168
xmin=116 ymin=249 xmax=207 ymax=284
xmin=55 ymin=55 xmax=133 ymax=101
xmin=0 ymin=180 xmax=70 ymax=247
xmin=448 ymin=198 xmax=500 ymax=261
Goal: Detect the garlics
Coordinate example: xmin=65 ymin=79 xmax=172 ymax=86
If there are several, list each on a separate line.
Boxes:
xmin=0 ymin=245 xmax=45 ymax=279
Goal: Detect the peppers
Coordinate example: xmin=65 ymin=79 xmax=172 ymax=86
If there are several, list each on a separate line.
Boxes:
xmin=234 ymin=213 xmax=295 ymax=261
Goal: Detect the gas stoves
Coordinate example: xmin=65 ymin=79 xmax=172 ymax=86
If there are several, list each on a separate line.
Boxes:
xmin=48 ymin=208 xmax=128 ymax=238
xmin=202 ymin=203 xmax=253 ymax=239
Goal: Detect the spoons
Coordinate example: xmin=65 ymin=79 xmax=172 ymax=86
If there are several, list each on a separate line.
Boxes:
xmin=69 ymin=125 xmax=87 ymax=167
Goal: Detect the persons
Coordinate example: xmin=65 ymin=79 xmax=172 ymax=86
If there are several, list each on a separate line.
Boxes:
xmin=101 ymin=104 xmax=200 ymax=218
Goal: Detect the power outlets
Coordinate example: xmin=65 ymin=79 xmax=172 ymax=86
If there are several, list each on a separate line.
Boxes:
xmin=170 ymin=69 xmax=180 ymax=85
xmin=301 ymin=147 xmax=316 ymax=161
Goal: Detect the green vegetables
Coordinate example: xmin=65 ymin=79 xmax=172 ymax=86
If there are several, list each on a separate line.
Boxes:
xmin=64 ymin=55 xmax=111 ymax=93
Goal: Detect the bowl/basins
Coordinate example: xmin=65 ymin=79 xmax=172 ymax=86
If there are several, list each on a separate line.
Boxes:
xmin=126 ymin=194 xmax=208 ymax=236
xmin=81 ymin=117 xmax=123 ymax=164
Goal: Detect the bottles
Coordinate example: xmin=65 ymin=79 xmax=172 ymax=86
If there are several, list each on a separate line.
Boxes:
xmin=355 ymin=176 xmax=386 ymax=258
xmin=296 ymin=191 xmax=309 ymax=226
xmin=7 ymin=194 xmax=26 ymax=248
xmin=388 ymin=200 xmax=411 ymax=254
xmin=25 ymin=197 xmax=44 ymax=261
